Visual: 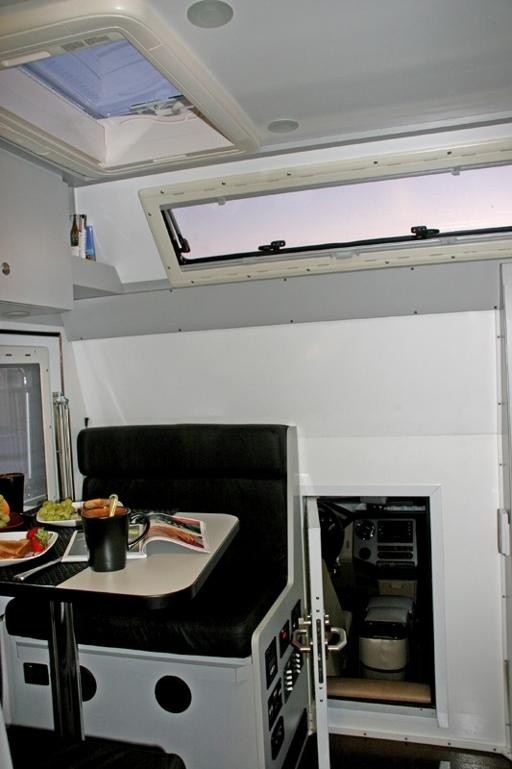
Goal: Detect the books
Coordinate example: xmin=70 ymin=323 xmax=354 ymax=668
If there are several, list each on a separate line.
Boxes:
xmin=61 ymin=511 xmax=212 ymax=564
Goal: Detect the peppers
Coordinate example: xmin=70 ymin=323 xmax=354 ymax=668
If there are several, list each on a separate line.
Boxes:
xmin=27 ymin=527 xmax=44 ymax=551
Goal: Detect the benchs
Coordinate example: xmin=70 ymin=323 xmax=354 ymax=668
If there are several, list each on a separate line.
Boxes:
xmin=0 ymin=424 xmax=311 ymax=769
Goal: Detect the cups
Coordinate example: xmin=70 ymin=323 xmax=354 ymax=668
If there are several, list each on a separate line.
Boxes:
xmin=0 ymin=473 xmax=25 ymax=510
xmin=85 ymin=508 xmax=148 ymax=574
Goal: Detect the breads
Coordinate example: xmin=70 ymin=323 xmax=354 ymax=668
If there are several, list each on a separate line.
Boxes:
xmin=0 ymin=539 xmax=30 ymax=558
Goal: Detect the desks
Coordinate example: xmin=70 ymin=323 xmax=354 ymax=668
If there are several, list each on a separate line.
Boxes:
xmin=2 ymin=500 xmax=240 ymax=769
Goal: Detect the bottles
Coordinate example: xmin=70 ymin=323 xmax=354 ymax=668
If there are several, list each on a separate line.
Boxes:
xmin=67 ymin=214 xmax=93 ymax=259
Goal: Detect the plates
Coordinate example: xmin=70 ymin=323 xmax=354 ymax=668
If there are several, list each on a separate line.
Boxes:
xmin=0 ymin=501 xmax=123 ymax=566
